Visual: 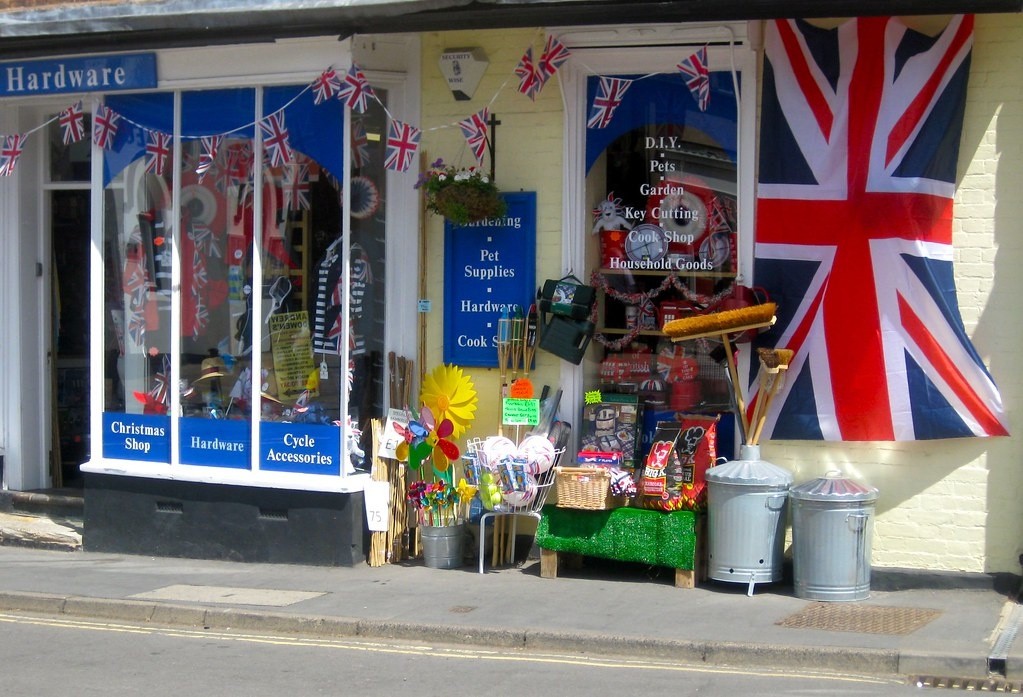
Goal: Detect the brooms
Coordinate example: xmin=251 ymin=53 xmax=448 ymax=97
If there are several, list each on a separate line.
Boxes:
xmin=662 ymin=302 xmax=794 ymax=445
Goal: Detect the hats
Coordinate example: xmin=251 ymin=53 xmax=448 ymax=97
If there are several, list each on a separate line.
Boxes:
xmin=191 ymin=357 xmax=232 ymax=388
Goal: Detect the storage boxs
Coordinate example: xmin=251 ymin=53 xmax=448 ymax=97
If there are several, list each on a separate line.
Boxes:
xmin=577 ymin=348 xmax=652 ymax=469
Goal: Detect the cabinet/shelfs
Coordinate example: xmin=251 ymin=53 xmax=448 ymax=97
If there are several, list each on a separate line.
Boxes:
xmin=593 ymin=270 xmax=738 ymax=410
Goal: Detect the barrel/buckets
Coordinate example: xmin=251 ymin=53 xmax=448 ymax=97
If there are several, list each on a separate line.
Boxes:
xmin=419 ymin=518 xmax=466 ymax=570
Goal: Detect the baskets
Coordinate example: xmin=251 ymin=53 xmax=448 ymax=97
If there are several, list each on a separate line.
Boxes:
xmin=467 ymin=437 xmax=567 ymax=515
xmin=552 ymin=467 xmax=631 ymax=511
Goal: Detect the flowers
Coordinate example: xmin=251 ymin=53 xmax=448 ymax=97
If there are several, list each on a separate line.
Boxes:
xmin=414 ymin=157 xmax=506 ymax=225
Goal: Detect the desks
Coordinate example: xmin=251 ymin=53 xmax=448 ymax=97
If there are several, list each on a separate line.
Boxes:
xmin=533 ymin=504 xmax=705 ymax=589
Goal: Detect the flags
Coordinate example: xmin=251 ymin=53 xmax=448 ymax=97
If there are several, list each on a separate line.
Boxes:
xmin=586 ymin=75 xmax=632 ymax=129
xmin=0 ymin=61 xmax=423 ymax=210
xmin=458 ymin=107 xmax=490 ymax=167
xmin=150 ymin=354 xmax=171 ymax=407
xmin=737 ymin=14 xmax=1010 ymax=442
xmin=675 ymin=45 xmax=710 ymax=111
xmin=514 ymin=34 xmax=572 ymax=102
